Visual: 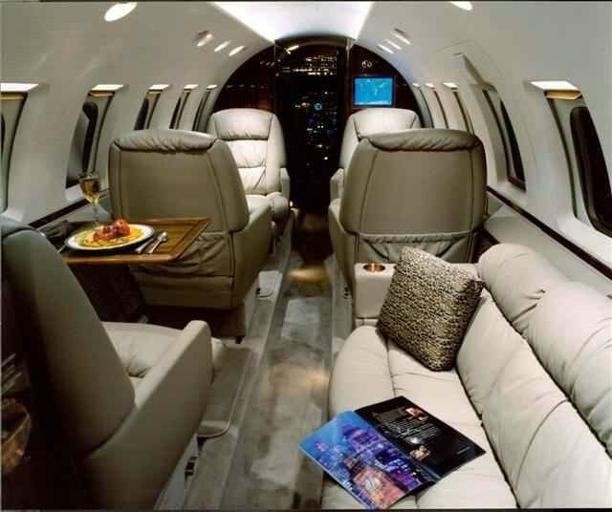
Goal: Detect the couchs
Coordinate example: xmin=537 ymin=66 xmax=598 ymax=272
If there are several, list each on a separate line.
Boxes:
xmin=314 ymin=234 xmax=612 ymax=509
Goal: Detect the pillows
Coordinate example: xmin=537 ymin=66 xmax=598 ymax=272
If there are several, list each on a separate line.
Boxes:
xmin=380 ymin=244 xmax=484 ymax=372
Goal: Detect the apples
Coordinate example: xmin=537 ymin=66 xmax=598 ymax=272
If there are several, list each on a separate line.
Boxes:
xmin=112 ymin=218 xmax=130 ymax=237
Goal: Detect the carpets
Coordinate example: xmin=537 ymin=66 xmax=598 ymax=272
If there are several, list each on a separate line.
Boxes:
xmin=259 ymin=253 xmax=283 ymax=296
xmin=195 ymin=344 xmax=253 ymax=438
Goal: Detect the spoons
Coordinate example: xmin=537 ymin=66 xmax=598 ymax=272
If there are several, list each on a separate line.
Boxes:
xmin=147 ymin=231 xmax=168 ymax=253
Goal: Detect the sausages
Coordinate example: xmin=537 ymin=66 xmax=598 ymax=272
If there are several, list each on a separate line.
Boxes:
xmin=92 ymin=224 xmax=117 ymax=243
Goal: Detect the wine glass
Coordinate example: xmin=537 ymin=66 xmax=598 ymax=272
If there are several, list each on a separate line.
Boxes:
xmin=79 ymin=171 xmax=101 ymax=225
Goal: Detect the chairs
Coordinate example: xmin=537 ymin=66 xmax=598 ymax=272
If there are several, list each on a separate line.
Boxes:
xmin=330 ymin=131 xmax=490 ymax=293
xmin=107 ymin=128 xmax=269 ymax=341
xmin=1 ymin=210 xmax=227 ymax=510
xmin=328 ymin=108 xmax=435 ymax=208
xmin=206 ymin=109 xmax=295 ymax=234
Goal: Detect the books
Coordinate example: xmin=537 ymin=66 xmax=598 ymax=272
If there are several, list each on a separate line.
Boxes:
xmin=297 ymin=395 xmax=486 ymax=512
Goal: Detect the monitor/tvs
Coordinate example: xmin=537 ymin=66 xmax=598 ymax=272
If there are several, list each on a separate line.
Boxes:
xmin=351 ymin=73 xmax=397 ymax=111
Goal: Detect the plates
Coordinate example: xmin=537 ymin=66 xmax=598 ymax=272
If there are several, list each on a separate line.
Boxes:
xmin=63 ymin=223 xmax=154 ymax=250
